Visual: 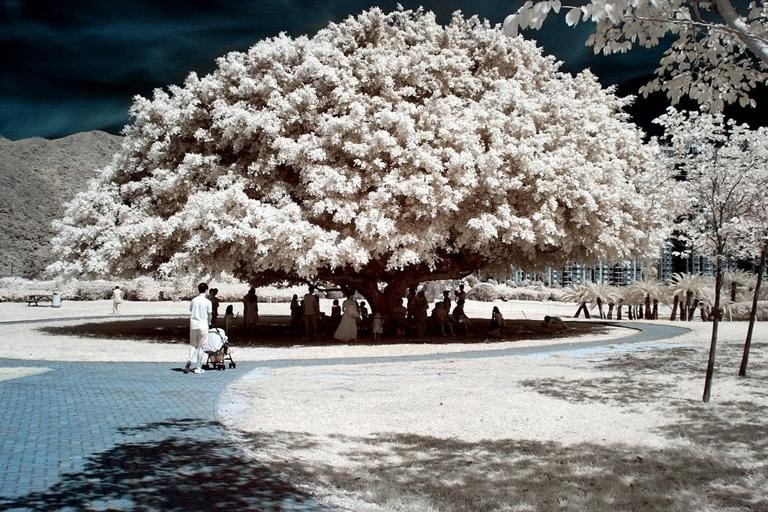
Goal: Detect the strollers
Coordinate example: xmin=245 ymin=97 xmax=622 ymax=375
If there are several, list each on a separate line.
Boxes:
xmin=203 ymin=320 xmax=235 ymax=371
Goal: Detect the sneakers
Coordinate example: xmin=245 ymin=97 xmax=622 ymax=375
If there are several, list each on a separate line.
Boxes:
xmin=184 ymin=361 xmax=191 ymax=373
xmin=194 ymin=369 xmax=205 ymax=374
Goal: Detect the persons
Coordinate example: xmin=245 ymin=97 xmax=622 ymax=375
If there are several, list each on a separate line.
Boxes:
xmin=223 ymin=304 xmax=237 ymax=339
xmin=540 ymin=315 xmax=568 ymax=330
xmin=242 ymin=290 xmax=250 ymax=326
xmin=110 ymin=286 xmax=122 ymax=313
xmin=243 ymin=286 xmax=258 ymax=334
xmin=206 ymin=287 xmax=220 ymax=328
xmin=183 ymin=282 xmax=212 ymax=375
xmin=50 ymin=290 xmax=61 ymax=308
xmin=288 ymin=284 xmax=476 ymax=346
xmin=490 ymin=306 xmax=507 ymax=327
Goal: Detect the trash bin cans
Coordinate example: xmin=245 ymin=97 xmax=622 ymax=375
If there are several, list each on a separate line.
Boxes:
xmin=52 ymin=290 xmax=61 ymax=307
